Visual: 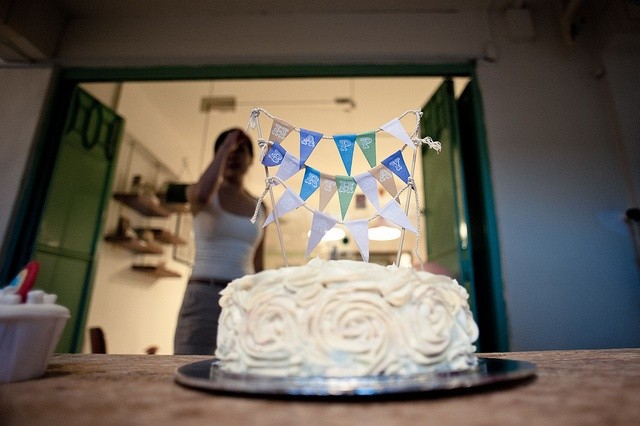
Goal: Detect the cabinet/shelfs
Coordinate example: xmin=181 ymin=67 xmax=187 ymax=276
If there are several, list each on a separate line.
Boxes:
xmin=102 ymin=194 xmax=187 ymax=277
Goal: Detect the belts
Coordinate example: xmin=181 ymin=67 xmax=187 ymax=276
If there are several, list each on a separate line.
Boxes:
xmin=187 ymin=278 xmax=229 ymax=289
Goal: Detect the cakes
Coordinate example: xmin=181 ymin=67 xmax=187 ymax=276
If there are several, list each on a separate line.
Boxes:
xmin=212 ymin=256 xmax=482 ymax=377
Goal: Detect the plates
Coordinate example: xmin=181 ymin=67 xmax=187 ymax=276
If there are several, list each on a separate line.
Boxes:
xmin=174 ymin=357 xmax=533 ymax=400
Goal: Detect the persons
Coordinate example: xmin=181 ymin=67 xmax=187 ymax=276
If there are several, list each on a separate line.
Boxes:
xmin=172 ymin=127 xmax=269 ymax=358
